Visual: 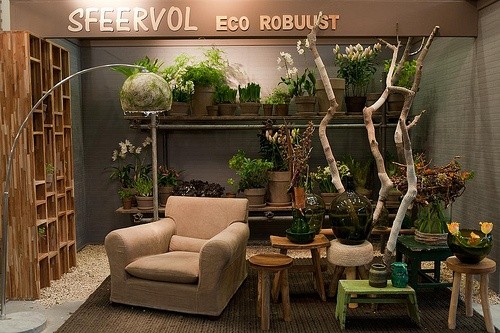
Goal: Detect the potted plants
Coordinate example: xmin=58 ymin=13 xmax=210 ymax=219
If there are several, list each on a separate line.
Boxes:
xmin=165 ymin=50 xmax=417 ymax=115
xmin=118 ymin=147 xmax=403 ymax=210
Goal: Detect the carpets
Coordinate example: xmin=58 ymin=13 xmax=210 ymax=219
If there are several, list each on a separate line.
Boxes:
xmin=53 ymin=256 xmax=500 ymax=333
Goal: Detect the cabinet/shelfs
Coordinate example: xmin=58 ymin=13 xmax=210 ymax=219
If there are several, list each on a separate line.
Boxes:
xmin=122 ymin=110 xmax=412 ymax=251
xmin=0 ymin=30 xmax=77 ymax=300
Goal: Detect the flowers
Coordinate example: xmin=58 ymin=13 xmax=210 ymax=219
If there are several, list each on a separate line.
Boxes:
xmin=103 ymin=135 xmax=152 ymax=187
xmin=158 ymin=164 xmax=184 ymax=187
xmin=333 ymin=42 xmax=381 ymax=96
xmin=446 ymin=220 xmax=494 ymax=249
xmin=314 ymin=158 xmax=352 ymax=193
xmin=159 ymin=52 xmax=196 ymax=101
xmin=275 ymin=39 xmax=319 ymax=97
xmin=266 ymin=128 xmax=300 ymax=171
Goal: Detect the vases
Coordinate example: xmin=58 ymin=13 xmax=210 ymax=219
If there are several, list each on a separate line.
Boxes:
xmin=167 ymin=102 xmax=190 ymax=115
xmin=292 ymin=186 xmax=374 ymax=245
xmin=344 ymin=95 xmax=367 ymax=113
xmin=295 ymin=96 xmax=315 ymax=115
xmin=447 ymin=230 xmax=494 ymax=265
xmin=267 ymin=171 xmax=294 ymax=206
xmin=158 ymin=186 xmax=176 ymax=208
xmin=314 ymin=78 xmax=346 ymax=112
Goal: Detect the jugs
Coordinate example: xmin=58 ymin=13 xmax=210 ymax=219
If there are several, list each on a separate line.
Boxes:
xmin=290 ymin=208 xmax=314 ymax=234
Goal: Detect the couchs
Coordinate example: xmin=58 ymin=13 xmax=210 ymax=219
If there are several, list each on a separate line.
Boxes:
xmin=104 ymin=195 xmax=250 ymax=321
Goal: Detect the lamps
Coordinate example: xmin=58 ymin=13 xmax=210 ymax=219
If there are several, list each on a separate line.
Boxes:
xmin=0 ymin=64 xmax=171 ymax=333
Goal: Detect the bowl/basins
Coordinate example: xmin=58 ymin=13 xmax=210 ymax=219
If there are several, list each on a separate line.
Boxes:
xmin=286 ymin=229 xmax=316 ymax=244
xmin=447 ymin=229 xmax=494 ymax=264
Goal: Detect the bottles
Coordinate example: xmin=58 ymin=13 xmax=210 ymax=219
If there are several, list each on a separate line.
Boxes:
xmin=389 ymin=262 xmax=410 ymax=288
xmin=295 ymin=176 xmax=324 ymax=233
xmin=369 ymin=264 xmax=387 ymax=288
xmin=330 ymin=175 xmax=373 ymax=245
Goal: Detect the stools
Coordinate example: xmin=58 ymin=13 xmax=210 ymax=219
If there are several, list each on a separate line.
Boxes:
xmin=247 ymin=228 xmax=497 ymax=333
